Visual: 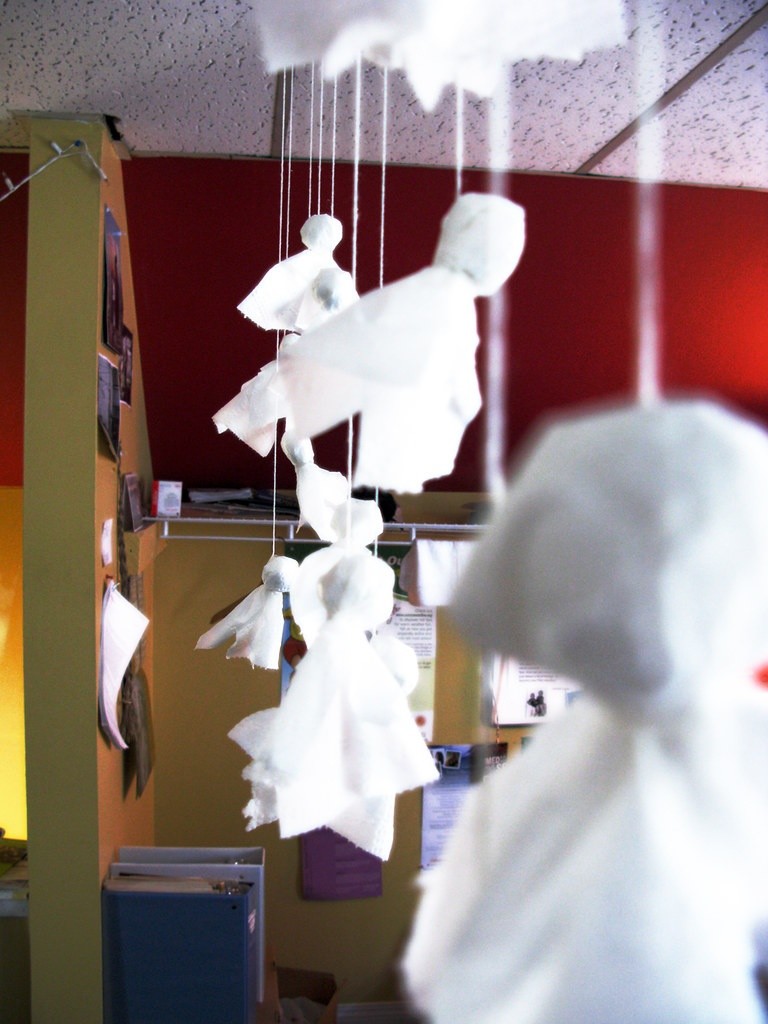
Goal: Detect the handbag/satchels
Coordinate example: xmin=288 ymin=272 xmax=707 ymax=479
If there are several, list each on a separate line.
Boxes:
xmin=273 ymin=966 xmax=339 ymax=1024
